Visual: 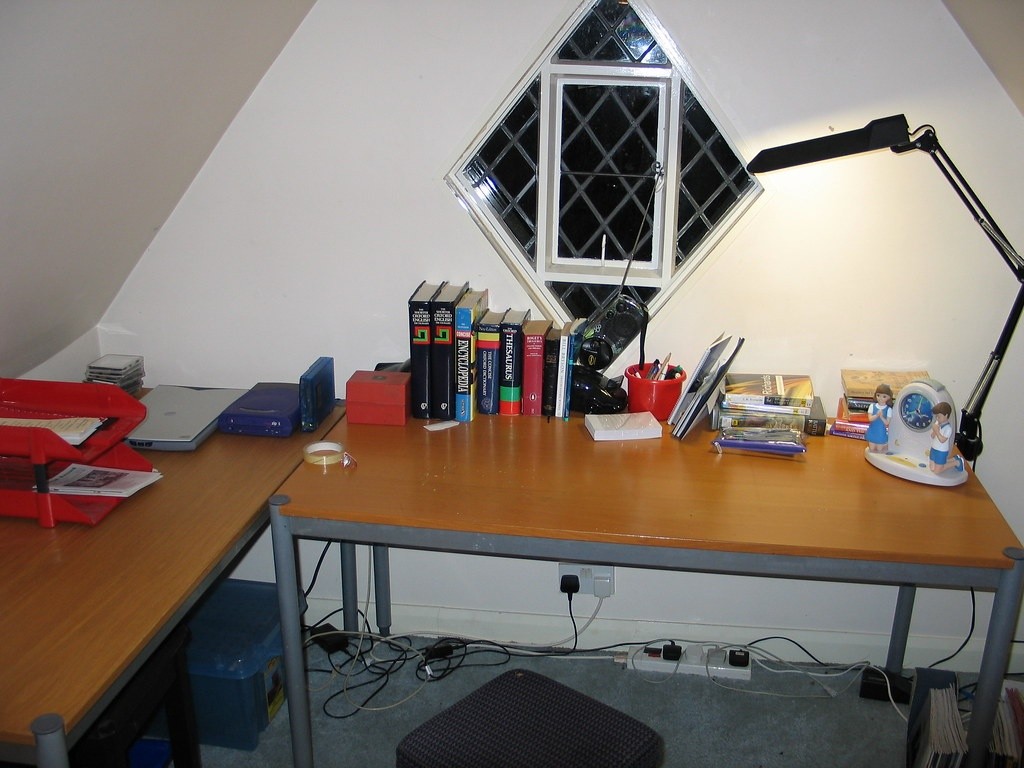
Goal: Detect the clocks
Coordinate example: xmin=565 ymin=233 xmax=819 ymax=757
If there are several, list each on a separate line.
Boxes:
xmin=864 ymin=378 xmax=969 ymax=486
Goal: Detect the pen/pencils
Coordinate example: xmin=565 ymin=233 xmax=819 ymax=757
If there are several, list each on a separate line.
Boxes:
xmin=633 ymin=351 xmax=683 ymax=380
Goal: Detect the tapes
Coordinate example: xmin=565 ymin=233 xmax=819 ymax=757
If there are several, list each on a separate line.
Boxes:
xmin=301 ymin=439 xmax=345 ymax=465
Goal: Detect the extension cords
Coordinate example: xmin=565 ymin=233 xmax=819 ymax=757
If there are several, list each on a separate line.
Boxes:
xmin=626 ymin=646 xmax=751 ymax=680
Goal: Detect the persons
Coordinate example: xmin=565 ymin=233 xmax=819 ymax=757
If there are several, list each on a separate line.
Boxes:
xmin=866 ymin=384 xmax=893 ymax=451
xmin=929 ymin=402 xmax=965 ymax=473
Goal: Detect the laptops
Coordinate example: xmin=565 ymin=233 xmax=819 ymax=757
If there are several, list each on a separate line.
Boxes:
xmin=123 ymin=384 xmax=250 ymax=451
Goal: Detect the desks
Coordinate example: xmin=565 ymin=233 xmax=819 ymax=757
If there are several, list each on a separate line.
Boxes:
xmin=266 ymin=408 xmax=1024 ymax=768
xmin=0 ymin=389 xmax=345 ymax=768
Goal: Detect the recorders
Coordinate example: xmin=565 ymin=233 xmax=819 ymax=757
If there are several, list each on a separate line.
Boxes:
xmin=576 ymin=294 xmax=651 ymax=374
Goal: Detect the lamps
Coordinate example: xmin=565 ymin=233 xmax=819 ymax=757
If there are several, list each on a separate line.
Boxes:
xmin=747 ymin=113 xmax=1024 ymax=460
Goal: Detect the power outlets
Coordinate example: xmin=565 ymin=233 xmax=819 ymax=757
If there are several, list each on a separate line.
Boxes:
xmin=558 ymin=563 xmax=614 ymax=594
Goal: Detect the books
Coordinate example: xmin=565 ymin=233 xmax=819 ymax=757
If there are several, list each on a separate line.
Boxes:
xmin=33 ymin=463 xmax=163 ymax=497
xmin=714 ymin=374 xmax=826 ymax=436
xmin=667 ymin=331 xmax=744 ymax=440
xmin=584 ymin=411 xmax=662 ymax=442
xmin=408 ymin=281 xmax=586 ymax=421
xmin=830 ymin=370 xmax=930 ymax=440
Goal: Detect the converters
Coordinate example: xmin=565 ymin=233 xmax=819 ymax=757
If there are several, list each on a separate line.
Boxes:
xmin=310 ymin=623 xmax=349 ymax=654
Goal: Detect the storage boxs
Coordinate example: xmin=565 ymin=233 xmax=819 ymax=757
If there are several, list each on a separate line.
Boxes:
xmin=345 ymin=370 xmax=411 ymax=426
xmin=219 ymin=382 xmax=299 ymax=437
xmin=299 ymin=356 xmax=336 ymax=432
xmin=146 ymin=578 xmax=307 ymax=751
